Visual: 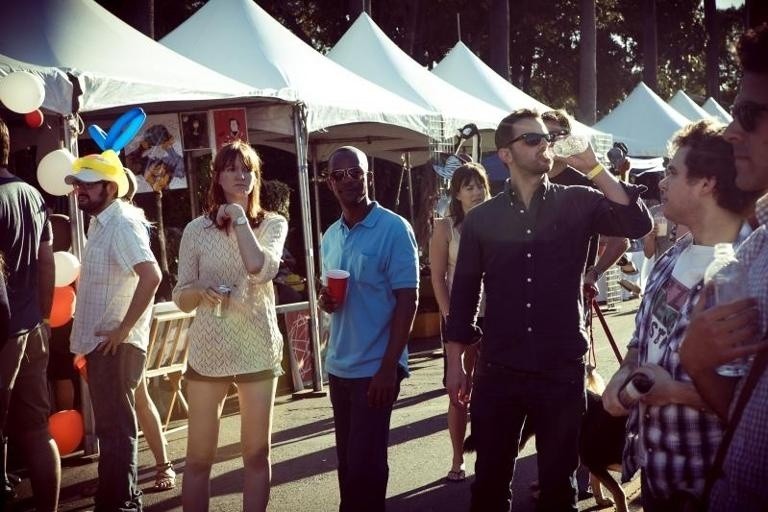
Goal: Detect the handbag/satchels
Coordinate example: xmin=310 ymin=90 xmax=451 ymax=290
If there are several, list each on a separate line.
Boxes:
xmin=665 ymin=333 xmax=768 ymax=512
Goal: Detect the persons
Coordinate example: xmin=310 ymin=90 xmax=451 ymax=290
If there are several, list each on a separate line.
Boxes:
xmin=122 ymin=167 xmax=176 ymax=493
xmin=0 ymin=120 xmax=64 ymax=512
xmin=431 ymin=161 xmax=492 ymax=480
xmin=319 ymin=147 xmax=420 ymax=512
xmin=681 ymin=22 xmax=768 ymax=512
xmin=641 ymin=202 xmax=693 ymax=264
xmin=602 ymin=123 xmax=757 ymax=512
xmin=445 ymin=109 xmax=652 ymax=511
xmin=63 ymin=155 xmax=163 ymax=512
xmin=172 ymin=142 xmax=289 ymax=512
xmin=541 ymin=111 xmax=634 ymax=299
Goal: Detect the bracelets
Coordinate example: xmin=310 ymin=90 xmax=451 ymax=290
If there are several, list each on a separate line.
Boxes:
xmin=585 ymin=164 xmax=603 ymax=180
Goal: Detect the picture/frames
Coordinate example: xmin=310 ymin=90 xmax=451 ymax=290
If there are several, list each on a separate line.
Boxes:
xmin=123 ymin=112 xmax=189 ymax=193
xmin=179 ymin=111 xmax=211 ymax=151
xmin=211 ymin=108 xmax=249 ymax=147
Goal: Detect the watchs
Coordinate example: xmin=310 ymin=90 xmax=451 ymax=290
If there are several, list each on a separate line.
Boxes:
xmin=231 ymin=216 xmax=247 ymax=227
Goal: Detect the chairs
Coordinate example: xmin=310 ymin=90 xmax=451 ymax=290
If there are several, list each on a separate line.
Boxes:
xmin=153 ymin=302 xmax=196 ymax=432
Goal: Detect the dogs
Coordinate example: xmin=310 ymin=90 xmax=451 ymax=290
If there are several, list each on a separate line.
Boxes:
xmin=462 ymin=387 xmax=639 ymax=512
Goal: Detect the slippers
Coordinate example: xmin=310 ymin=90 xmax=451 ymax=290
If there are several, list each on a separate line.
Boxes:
xmin=447 ymin=467 xmax=466 ymax=483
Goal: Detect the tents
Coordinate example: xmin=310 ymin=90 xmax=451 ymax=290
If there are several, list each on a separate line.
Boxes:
xmin=593 ymin=81 xmax=694 ymax=159
xmin=668 ymin=91 xmax=718 ymax=124
xmin=701 ymin=97 xmax=734 ymax=126
xmin=324 ymin=12 xmax=511 ymax=237
xmin=0 ymin=1 xmax=318 ymax=454
xmin=0 ymin=56 xmax=94 ymax=455
xmin=156 ymin=0 xmax=441 ymax=391
xmin=431 ymin=41 xmax=615 ymax=158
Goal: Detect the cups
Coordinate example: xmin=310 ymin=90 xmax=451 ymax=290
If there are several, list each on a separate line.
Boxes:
xmin=323 ymin=270 xmax=349 ymax=313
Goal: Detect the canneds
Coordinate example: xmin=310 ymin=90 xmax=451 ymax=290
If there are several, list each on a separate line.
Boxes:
xmin=211 ymin=284 xmax=231 ymax=319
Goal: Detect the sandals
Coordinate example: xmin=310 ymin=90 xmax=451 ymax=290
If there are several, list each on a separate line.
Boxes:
xmin=154 ymin=461 xmax=178 ymax=492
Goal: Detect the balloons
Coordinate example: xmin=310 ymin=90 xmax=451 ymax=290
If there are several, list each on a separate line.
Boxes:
xmin=53 ymin=252 xmax=79 ymax=287
xmin=0 ymin=72 xmax=45 ymax=113
xmin=37 ymin=148 xmax=79 ymax=196
xmin=73 ymin=107 xmax=146 ymax=199
xmin=48 ymin=286 xmax=76 ymax=328
xmin=48 ymin=408 xmax=82 ymax=454
xmin=24 ymin=110 xmax=43 ymax=127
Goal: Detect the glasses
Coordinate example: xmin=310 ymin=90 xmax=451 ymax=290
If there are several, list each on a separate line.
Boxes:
xmin=73 ymin=182 xmax=93 ymax=191
xmin=505 ymin=132 xmax=556 ymax=146
xmin=328 ymin=166 xmax=368 ymax=181
xmin=729 ymin=101 xmax=768 ymax=131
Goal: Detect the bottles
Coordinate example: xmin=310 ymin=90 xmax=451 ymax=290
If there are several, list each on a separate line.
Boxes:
xmin=704 ymin=242 xmax=756 ymax=377
xmin=542 ymin=134 xmax=589 ymax=159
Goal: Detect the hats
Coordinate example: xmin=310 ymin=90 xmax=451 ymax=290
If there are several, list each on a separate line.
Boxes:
xmin=621 ymin=261 xmax=639 ymax=275
xmin=617 ymin=279 xmax=634 ymax=292
xmin=631 ymin=284 xmax=640 ymax=298
xmin=616 ymin=158 xmax=629 ymax=174
xmin=607 ymin=147 xmax=625 ymax=165
xmin=433 ymin=154 xmax=470 ymax=181
xmin=64 ymin=155 xmax=128 ymax=200
xmin=613 ymin=141 xmax=628 ymax=154
xmin=616 ymin=254 xmax=628 ymax=266
